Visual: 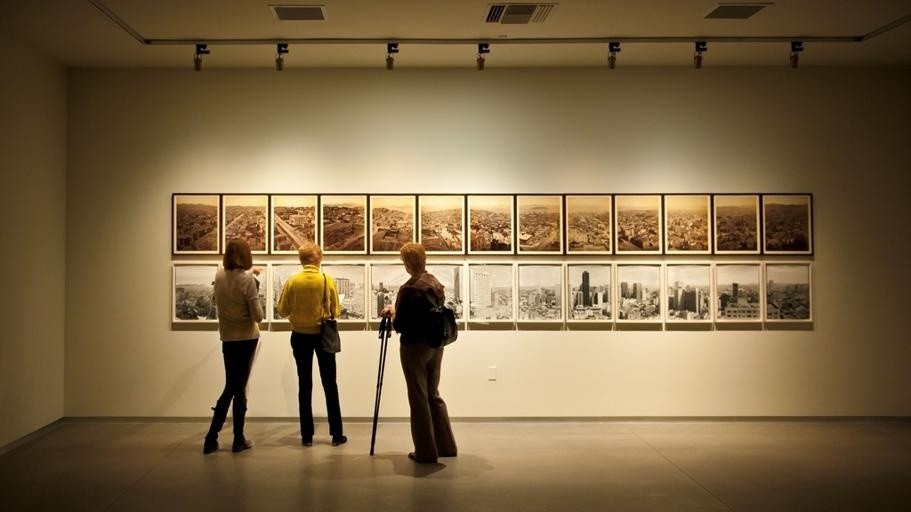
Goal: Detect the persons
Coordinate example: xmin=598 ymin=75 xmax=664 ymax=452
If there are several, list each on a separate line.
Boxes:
xmin=201 ymin=238 xmax=265 ymax=454
xmin=275 ymin=242 xmax=349 ymax=447
xmin=380 ymin=240 xmax=461 ymax=464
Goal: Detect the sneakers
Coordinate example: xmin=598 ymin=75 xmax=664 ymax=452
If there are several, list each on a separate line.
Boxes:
xmin=304 ymin=438 xmax=312 ymax=447
xmin=332 ymin=435 xmax=346 ymax=445
xmin=408 ymin=452 xmax=439 ymax=462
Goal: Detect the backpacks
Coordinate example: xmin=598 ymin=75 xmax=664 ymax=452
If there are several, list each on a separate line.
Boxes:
xmin=425 ymin=296 xmax=458 ymax=349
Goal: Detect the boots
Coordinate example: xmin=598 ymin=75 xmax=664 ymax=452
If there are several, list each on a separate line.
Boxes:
xmin=204 ymin=400 xmax=229 ymax=454
xmin=232 ymin=397 xmax=253 ymax=452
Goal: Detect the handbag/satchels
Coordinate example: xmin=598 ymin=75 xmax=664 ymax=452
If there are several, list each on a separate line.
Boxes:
xmin=320 ymin=317 xmax=340 ymax=354
